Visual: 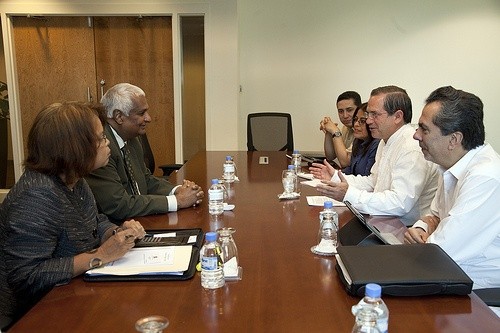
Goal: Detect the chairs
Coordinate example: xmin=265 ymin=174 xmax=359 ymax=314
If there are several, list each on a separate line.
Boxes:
xmin=140 ymin=131 xmax=183 ymax=177
xmin=247 ymin=112 xmax=294 ymax=151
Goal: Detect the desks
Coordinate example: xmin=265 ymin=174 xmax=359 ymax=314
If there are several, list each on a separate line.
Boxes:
xmin=5 ymin=150 xmax=500 ymax=333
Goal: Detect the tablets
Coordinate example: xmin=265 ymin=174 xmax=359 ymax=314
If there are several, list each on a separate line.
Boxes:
xmin=344 ymin=200 xmax=402 ymax=246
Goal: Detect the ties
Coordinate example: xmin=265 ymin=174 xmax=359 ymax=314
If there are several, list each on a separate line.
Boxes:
xmin=120 ymin=143 xmax=140 ymax=195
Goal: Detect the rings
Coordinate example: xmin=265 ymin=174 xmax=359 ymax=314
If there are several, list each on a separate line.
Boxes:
xmin=430 ymin=218 xmax=432 ymax=221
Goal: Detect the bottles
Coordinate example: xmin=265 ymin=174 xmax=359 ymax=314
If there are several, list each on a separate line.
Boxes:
xmin=287 ymin=165 xmax=298 ymax=190
xmin=223 ymin=155 xmax=236 ymax=183
xmin=208 ymin=178 xmax=225 ymax=215
xmin=290 ymin=151 xmax=302 ymax=174
xmin=318 ymin=201 xmax=339 ymax=241
xmin=200 ymin=232 xmax=226 ymax=289
xmin=355 ymin=283 xmax=389 ymax=333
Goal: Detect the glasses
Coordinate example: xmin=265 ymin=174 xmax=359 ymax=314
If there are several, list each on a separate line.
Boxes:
xmin=365 ymin=112 xmax=387 ymax=119
xmin=353 ymin=117 xmax=366 ymax=126
xmin=98 ymin=135 xmax=107 ymax=143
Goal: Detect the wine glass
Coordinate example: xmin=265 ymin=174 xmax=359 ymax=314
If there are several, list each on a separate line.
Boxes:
xmin=229 ymin=157 xmax=238 ymax=179
xmin=217 ymin=226 xmax=240 ymax=279
xmin=216 ymin=178 xmax=229 ymax=208
xmin=315 ymin=212 xmax=341 ymax=253
xmin=291 ymin=153 xmax=302 ymax=173
xmin=281 ymin=170 xmax=297 ymax=198
xmin=135 ymin=315 xmax=169 ymax=333
xmin=352 ymin=296 xmax=385 ymax=333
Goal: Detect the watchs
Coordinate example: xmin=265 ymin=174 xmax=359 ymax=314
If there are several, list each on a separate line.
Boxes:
xmin=332 ymin=131 xmax=342 ymax=139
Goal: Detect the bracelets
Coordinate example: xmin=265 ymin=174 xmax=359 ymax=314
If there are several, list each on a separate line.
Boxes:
xmin=86 ymin=248 xmax=103 ymax=269
xmin=112 ymin=226 xmax=122 ymax=236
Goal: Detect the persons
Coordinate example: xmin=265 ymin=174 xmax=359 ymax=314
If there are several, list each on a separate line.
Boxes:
xmin=319 ymin=91 xmax=362 ymax=168
xmin=308 ymin=85 xmax=443 ymax=228
xmin=0 ymin=100 xmax=148 ymax=333
xmin=83 ymin=82 xmax=205 ymax=225
xmin=340 ymin=101 xmax=381 ymax=178
xmin=403 ymin=85 xmax=500 ymax=318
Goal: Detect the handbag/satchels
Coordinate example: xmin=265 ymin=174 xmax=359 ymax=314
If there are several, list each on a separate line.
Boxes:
xmin=334 ymin=243 xmax=474 ymax=297
